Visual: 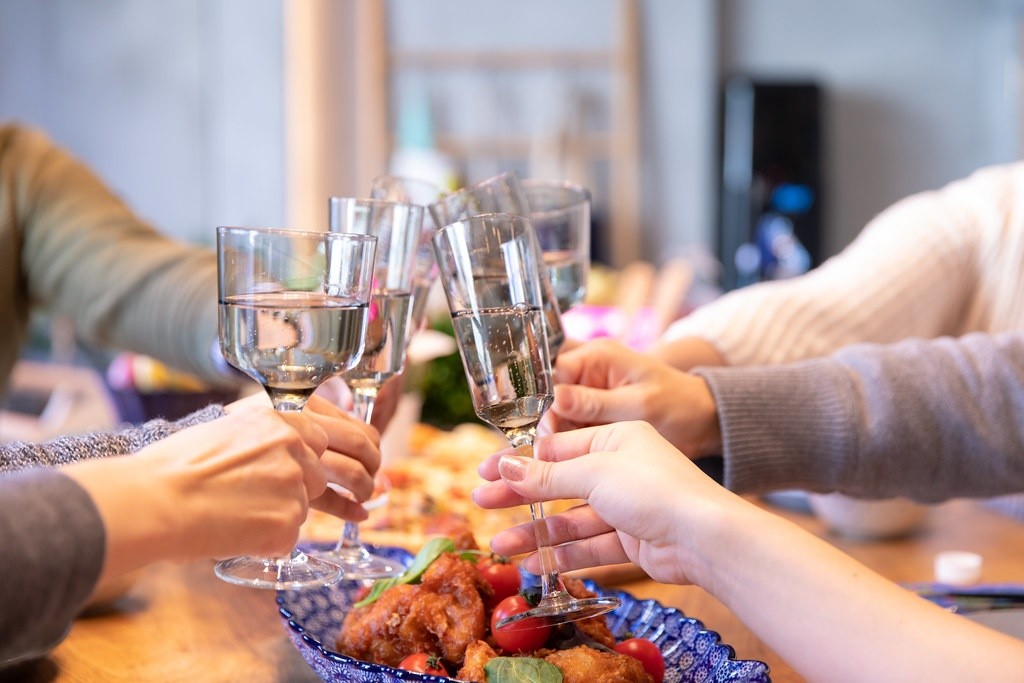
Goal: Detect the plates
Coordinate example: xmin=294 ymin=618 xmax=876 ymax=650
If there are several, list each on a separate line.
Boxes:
xmin=283 ymin=543 xmax=773 ymax=683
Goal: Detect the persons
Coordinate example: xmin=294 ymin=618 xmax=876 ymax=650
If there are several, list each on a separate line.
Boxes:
xmin=535 ymin=325 xmax=1024 ymax=504
xmin=0 ymin=405 xmax=328 ymax=667
xmin=1 ymin=122 xmax=398 ymax=434
xmin=473 ymin=419 xmax=1024 ymax=683
xmin=0 ymin=388 xmax=382 ymax=520
xmin=557 ymin=160 xmax=1024 ymax=372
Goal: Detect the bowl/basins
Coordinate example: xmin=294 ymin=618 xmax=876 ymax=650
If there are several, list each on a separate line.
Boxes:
xmin=813 ymin=493 xmax=922 ymax=538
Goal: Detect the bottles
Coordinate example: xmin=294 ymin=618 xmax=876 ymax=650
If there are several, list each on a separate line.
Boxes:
xmin=759 ymin=187 xmax=814 ymax=279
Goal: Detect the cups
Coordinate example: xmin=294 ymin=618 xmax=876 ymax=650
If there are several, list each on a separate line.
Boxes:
xmin=522 ymin=178 xmax=592 ymax=313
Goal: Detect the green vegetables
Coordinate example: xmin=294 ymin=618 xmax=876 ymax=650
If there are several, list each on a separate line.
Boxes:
xmin=482 ymin=657 xmax=564 ymax=683
xmin=352 ymin=537 xmax=478 ymax=610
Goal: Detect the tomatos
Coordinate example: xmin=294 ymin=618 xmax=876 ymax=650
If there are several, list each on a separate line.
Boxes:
xmin=612 ymin=637 xmax=664 ymax=683
xmin=397 ymin=653 xmax=450 ymax=677
xmin=478 ymin=554 xmax=523 ymax=599
xmin=490 ymin=595 xmax=552 ymax=654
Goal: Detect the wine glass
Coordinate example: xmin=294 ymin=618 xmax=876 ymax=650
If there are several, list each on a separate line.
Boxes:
xmin=367 ymin=175 xmax=445 ymax=504
xmin=315 ymin=196 xmax=424 ymax=578
xmin=213 ymin=226 xmax=379 ymax=589
xmin=431 ymin=212 xmax=621 ymax=632
xmin=429 ymin=173 xmax=582 ymax=431
